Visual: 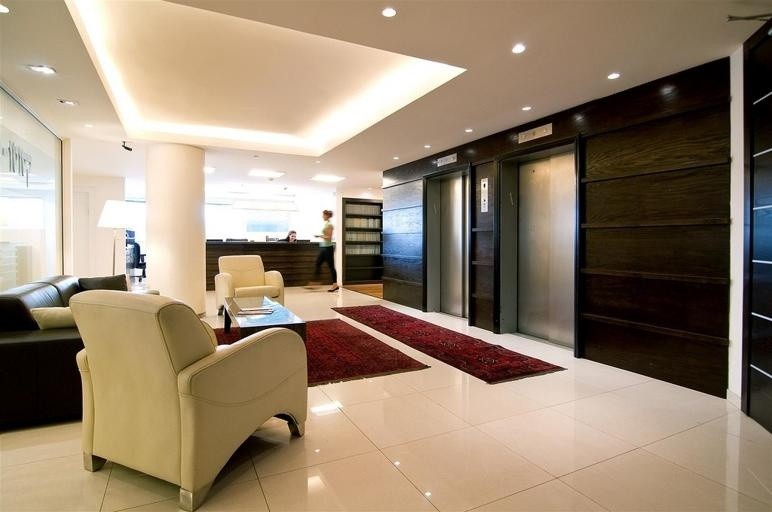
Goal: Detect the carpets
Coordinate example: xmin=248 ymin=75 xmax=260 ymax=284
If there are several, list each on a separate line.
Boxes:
xmin=214 ymin=318 xmax=431 ymax=386
xmin=332 ymin=305 xmax=566 ymax=385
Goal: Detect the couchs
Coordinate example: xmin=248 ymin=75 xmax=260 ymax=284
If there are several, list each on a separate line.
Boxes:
xmin=0 ymin=275 xmax=160 ymax=433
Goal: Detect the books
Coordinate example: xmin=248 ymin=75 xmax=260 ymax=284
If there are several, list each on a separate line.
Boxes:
xmin=345 ymin=204 xmax=381 ymax=254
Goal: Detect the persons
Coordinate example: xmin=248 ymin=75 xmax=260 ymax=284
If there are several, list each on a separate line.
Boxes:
xmin=305 ymin=209 xmax=340 ymax=292
xmin=287 ymin=230 xmax=297 ymax=242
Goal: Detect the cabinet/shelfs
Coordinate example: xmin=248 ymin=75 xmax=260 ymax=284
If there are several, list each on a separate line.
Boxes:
xmin=342 ymin=197 xmax=383 ymax=285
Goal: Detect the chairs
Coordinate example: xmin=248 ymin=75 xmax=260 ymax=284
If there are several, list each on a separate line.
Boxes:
xmin=68 ymin=288 xmax=308 ymax=511
xmin=214 ymin=254 xmax=285 ymax=315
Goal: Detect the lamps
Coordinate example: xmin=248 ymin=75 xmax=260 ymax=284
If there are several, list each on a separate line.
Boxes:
xmin=97 ymin=199 xmax=146 ymax=276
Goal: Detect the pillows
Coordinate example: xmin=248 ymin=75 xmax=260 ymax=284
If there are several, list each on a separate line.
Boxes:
xmin=78 ymin=273 xmax=128 ymax=291
xmin=30 ymin=306 xmax=77 ymax=330
